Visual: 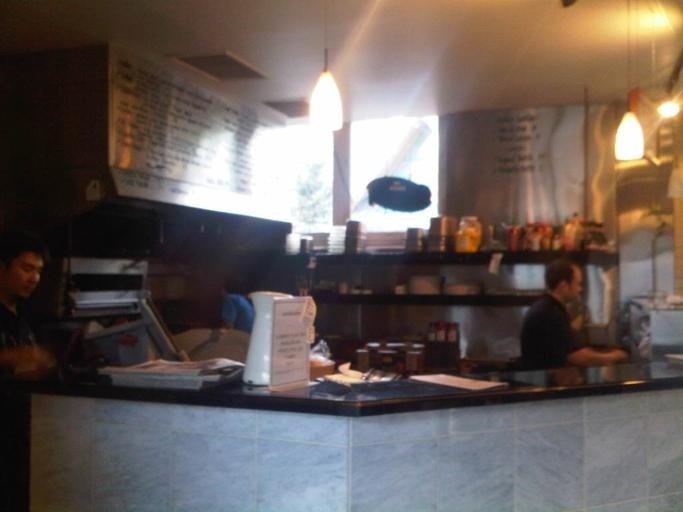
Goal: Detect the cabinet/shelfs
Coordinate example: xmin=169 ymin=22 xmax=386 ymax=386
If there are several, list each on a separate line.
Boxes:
xmin=285 ymin=248 xmax=617 ymax=375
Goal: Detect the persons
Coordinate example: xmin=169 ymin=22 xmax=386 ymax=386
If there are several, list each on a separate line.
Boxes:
xmin=219 ymin=269 xmax=255 ymax=334
xmin=520 ymin=260 xmax=628 ymax=371
xmin=0 ymin=232 xmax=57 ymax=380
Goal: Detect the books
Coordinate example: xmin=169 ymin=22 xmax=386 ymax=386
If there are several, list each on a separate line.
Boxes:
xmin=96 ymin=359 xmax=243 ymax=393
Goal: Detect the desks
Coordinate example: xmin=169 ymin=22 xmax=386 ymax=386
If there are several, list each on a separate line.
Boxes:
xmin=21 ymin=360 xmax=683 ymax=512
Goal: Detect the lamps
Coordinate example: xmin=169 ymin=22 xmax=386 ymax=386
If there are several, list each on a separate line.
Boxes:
xmin=308 ymin=0 xmax=343 ymax=132
xmin=614 ymin=0 xmax=644 ymax=161
